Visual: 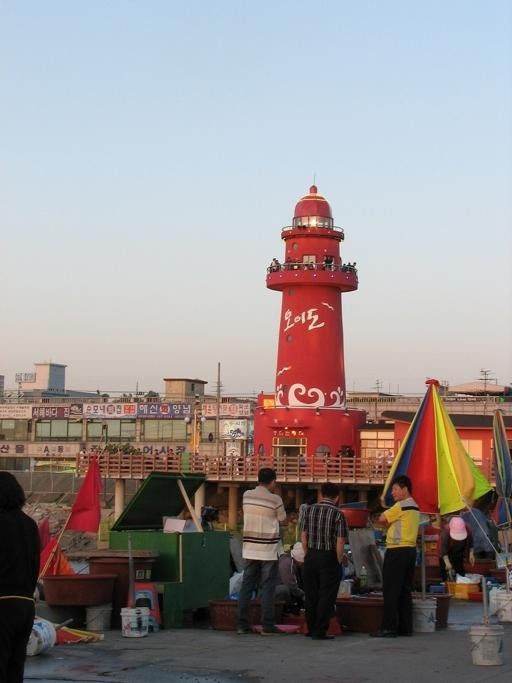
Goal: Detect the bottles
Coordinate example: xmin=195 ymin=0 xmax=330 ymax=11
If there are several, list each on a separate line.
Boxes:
xmin=359 ymin=566 xmax=368 ymax=594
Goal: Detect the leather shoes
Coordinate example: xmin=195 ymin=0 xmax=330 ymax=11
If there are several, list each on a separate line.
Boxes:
xmin=237 ymin=622 xmax=414 ymax=640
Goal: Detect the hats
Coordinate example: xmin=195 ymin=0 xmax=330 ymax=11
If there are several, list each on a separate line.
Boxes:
xmin=448 ymin=516 xmax=467 ymax=541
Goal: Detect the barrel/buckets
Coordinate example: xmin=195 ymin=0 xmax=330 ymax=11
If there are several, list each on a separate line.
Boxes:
xmin=467 ymin=622 xmax=506 ymax=664
xmin=495 ymin=590 xmax=511 ymax=622
xmin=120 ymin=605 xmax=152 ymax=637
xmin=412 ymin=599 xmax=439 ymax=633
xmin=84 ymin=605 xmax=114 ymax=631
xmin=28 ymin=621 xmax=57 ymax=656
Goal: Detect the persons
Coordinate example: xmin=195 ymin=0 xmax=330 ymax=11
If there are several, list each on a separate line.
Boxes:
xmin=237 ymin=466 xmax=289 ymax=637
xmin=299 ymin=481 xmax=348 ymax=641
xmin=269 ymin=256 xmax=357 ymax=273
xmin=369 ymin=474 xmax=422 ymax=638
xmin=274 ymin=540 xmax=305 ymax=616
xmin=78 ymin=447 xmax=356 ymax=480
xmin=436 ymin=516 xmax=475 ymax=581
xmin=0 ymin=470 xmax=43 ymax=683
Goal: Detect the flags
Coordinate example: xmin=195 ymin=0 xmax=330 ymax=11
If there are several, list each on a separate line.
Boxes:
xmin=66 ymin=460 xmax=103 ymax=533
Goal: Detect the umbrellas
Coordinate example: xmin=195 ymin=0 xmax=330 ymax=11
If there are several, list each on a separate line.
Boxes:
xmin=378 ymin=377 xmax=494 ymax=600
xmin=492 ymin=404 xmax=512 ymax=553
